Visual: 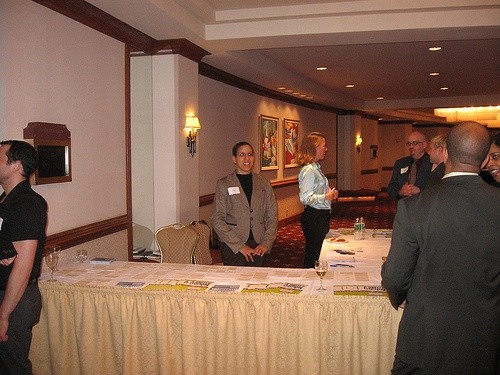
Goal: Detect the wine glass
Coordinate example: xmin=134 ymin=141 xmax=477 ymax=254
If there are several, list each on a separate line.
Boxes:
xmin=45 ymin=253 xmax=58 ymax=282
xmin=315 ymin=260 xmax=328 ymax=291
xmin=50 ymin=246 xmax=61 ymax=271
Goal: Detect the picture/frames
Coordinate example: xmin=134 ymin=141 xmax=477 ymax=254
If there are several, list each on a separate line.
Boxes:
xmin=260 ymin=114 xmax=280 ymax=171
xmin=283 ymin=118 xmax=301 ymax=169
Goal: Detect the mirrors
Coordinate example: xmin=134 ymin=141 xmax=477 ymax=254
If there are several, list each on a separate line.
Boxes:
xmin=30 ymin=137 xmax=72 ymax=186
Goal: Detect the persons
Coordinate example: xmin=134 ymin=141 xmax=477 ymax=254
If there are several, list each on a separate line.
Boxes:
xmin=298 ymin=132 xmax=339 ymax=269
xmin=420 ymin=134 xmax=448 ymax=190
xmin=486 ymin=132 xmax=500 ymax=182
xmin=211 ymin=142 xmax=278 ymax=267
xmin=381 ymin=121 xmax=500 ymax=375
xmin=387 ymin=130 xmax=427 ymax=203
xmin=0 ymin=139 xmax=47 ymax=375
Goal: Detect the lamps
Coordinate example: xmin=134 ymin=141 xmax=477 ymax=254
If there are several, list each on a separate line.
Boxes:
xmin=184 ymin=116 xmax=201 ymax=158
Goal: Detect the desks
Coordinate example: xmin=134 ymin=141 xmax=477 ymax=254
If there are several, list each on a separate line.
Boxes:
xmin=318 ymin=229 xmax=393 ymax=266
xmin=29 ymin=261 xmax=405 ymax=375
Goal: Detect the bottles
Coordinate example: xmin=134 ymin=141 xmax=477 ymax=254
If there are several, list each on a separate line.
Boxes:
xmin=360 ymin=216 xmax=366 ymax=240
xmin=354 ymin=217 xmax=360 ymax=240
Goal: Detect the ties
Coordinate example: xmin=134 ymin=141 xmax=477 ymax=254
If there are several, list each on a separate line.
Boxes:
xmin=407 ymin=158 xmax=419 ymax=185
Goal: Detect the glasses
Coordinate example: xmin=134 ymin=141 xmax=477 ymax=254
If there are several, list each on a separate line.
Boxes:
xmin=406 ymin=141 xmax=424 ymax=146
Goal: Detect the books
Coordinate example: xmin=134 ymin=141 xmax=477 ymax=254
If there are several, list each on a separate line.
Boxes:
xmin=90 ymin=257 xmax=116 ymax=264
xmin=133 ymin=248 xmax=161 ymax=258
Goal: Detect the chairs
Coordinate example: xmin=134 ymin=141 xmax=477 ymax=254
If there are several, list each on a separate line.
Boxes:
xmin=154 ymin=223 xmax=201 ymax=264
xmin=189 ymin=220 xmax=224 ymax=265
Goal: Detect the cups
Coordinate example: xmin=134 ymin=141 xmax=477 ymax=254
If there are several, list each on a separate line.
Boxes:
xmin=75 ymin=250 xmax=87 ymax=262
